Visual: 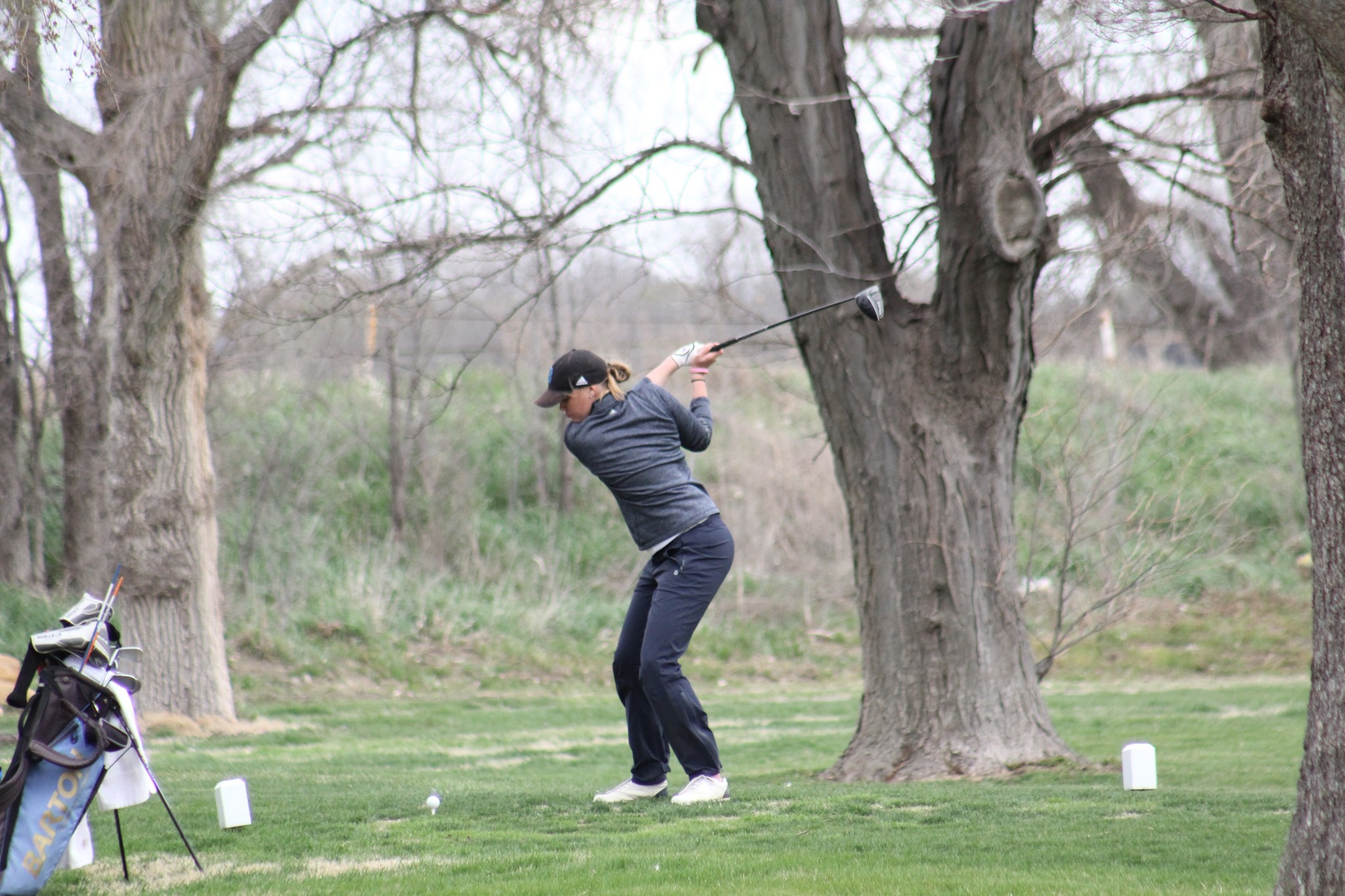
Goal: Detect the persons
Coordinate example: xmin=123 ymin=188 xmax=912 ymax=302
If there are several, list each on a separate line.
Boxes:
xmin=536 ymin=343 xmax=735 ymax=807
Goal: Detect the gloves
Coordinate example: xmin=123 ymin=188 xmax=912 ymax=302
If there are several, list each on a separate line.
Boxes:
xmin=671 ymin=341 xmax=707 ymax=369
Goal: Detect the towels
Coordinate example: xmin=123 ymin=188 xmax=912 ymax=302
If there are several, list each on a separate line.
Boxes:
xmin=55 ymin=654 xmax=157 ymax=870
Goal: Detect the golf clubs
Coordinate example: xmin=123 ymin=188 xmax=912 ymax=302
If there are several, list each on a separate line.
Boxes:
xmin=709 ymin=284 xmax=885 ymax=353
xmin=104 ymin=674 xmax=141 ymax=695
xmin=100 ymin=646 xmax=143 ymax=686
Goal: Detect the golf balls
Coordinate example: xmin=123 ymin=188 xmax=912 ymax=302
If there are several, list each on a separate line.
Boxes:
xmin=426 ymin=796 xmax=440 ymax=808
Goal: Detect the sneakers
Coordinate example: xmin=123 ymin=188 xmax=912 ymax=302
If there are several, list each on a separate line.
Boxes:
xmin=671 ymin=774 xmax=730 ymax=805
xmin=593 ymin=776 xmax=668 ymax=804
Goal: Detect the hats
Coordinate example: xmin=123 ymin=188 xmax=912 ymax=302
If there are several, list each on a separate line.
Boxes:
xmin=534 ymin=348 xmax=608 ymax=408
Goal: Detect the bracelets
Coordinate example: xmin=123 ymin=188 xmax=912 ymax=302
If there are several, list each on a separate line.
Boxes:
xmin=690 ymin=369 xmax=708 ymax=373
xmin=691 ymin=379 xmax=705 ymax=382
